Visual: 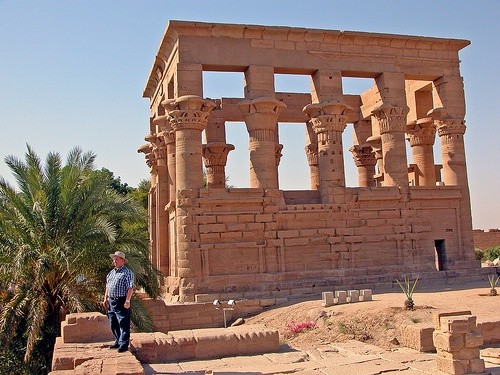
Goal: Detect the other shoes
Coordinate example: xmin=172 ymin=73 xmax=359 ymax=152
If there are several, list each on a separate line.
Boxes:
xmin=110 ymin=343 xmax=119 ymax=348
xmin=119 ymin=345 xmax=128 ymax=352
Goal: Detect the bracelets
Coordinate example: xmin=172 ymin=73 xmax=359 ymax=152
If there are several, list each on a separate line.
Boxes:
xmin=125 ymin=300 xmax=130 ymax=303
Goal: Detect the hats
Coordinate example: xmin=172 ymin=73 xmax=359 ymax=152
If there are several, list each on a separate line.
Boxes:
xmin=110 ymin=251 xmax=125 ymax=260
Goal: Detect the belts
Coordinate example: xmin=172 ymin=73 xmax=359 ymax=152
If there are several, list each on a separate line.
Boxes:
xmin=109 ymin=297 xmax=126 ymax=300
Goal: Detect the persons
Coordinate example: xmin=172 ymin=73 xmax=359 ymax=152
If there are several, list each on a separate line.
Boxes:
xmin=102 ymin=251 xmax=134 ymax=353
